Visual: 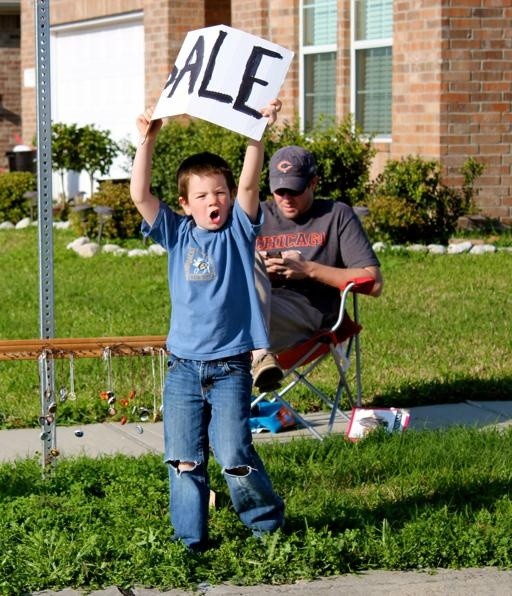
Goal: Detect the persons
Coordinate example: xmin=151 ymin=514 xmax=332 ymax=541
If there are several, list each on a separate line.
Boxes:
xmin=129 ymin=99 xmax=284 ymax=555
xmin=247 ymin=145 xmax=384 ymax=392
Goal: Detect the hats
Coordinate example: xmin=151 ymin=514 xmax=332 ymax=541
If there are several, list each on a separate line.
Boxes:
xmin=268 ymin=147 xmax=315 ymax=193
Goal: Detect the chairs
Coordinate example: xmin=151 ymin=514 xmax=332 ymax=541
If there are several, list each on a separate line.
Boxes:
xmin=248 ymin=205 xmax=378 ymax=442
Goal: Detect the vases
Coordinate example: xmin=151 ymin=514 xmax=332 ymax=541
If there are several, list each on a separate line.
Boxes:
xmin=6 ymin=149 xmax=36 ymax=173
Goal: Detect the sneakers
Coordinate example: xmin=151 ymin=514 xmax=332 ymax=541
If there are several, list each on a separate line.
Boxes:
xmin=252 ymin=353 xmax=283 ymax=393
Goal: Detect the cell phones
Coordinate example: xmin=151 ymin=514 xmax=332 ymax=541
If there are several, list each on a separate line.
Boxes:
xmin=266 ymin=248 xmax=282 ymax=258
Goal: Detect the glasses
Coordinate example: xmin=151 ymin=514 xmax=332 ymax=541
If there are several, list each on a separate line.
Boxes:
xmin=275 ymin=188 xmax=305 ymax=197
